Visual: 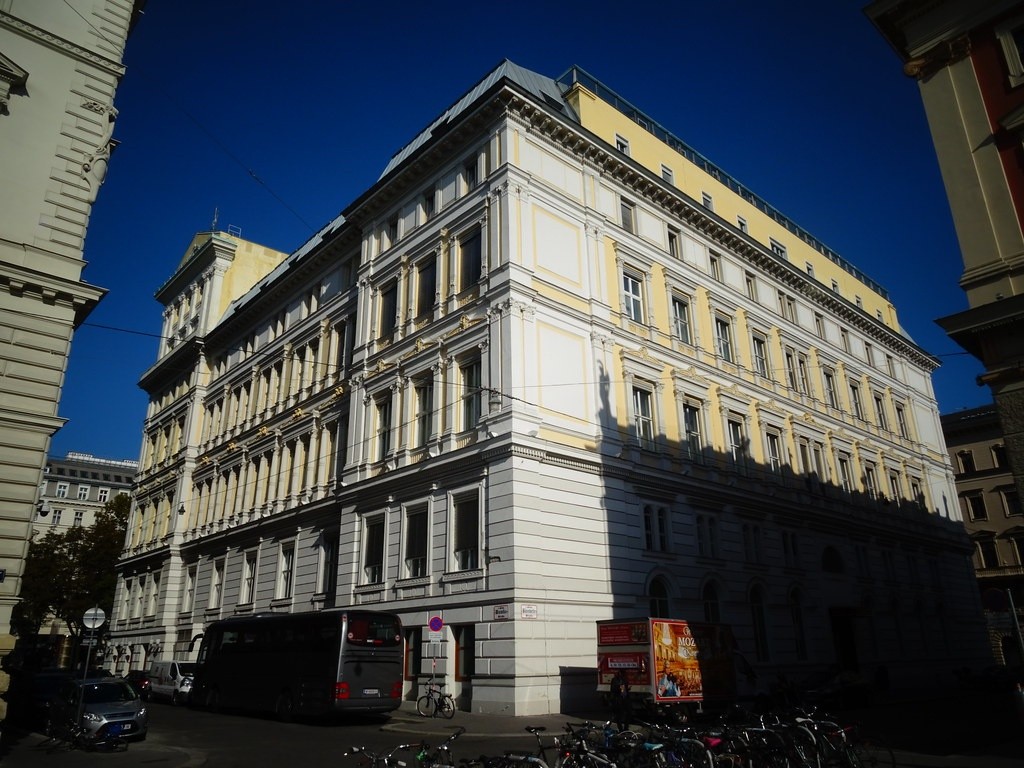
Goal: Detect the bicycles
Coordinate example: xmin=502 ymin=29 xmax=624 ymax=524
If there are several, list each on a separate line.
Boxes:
xmin=343 ymin=699 xmax=897 ymax=767
xmin=417 ymin=678 xmax=456 ymax=720
xmin=39 ymin=720 xmax=129 ymax=753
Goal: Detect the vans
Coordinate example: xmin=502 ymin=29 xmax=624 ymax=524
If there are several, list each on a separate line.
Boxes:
xmin=146 ymin=661 xmax=204 ymax=707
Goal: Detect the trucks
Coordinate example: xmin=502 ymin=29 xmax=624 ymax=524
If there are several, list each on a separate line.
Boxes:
xmin=594 ymin=615 xmax=777 ymax=728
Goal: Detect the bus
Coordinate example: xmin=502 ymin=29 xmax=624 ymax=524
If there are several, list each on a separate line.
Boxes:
xmin=187 ymin=609 xmax=405 ymax=724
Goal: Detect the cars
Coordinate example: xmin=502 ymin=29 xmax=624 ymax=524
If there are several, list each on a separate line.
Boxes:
xmin=119 ymin=670 xmax=152 ymax=701
xmin=54 ymin=676 xmax=148 ymax=748
xmin=87 ymin=669 xmax=115 ymax=697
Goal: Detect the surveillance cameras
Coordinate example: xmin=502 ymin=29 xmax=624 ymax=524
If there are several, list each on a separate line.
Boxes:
xmin=39 ymin=501 xmax=50 ymax=517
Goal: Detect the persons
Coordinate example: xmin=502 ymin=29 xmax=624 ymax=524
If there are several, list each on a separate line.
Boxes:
xmin=611 ymin=669 xmax=632 ymax=733
